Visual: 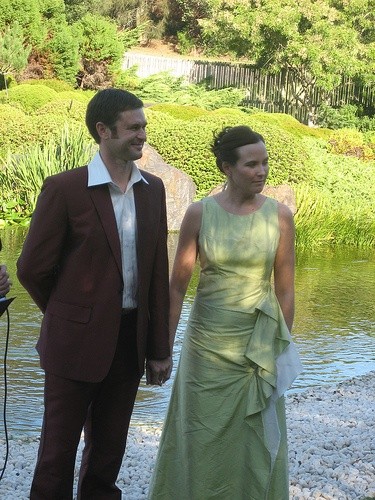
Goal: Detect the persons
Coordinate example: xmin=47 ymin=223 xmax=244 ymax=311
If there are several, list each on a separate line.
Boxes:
xmin=16 ymin=89 xmax=172 ymax=500
xmin=152 ymin=126 xmax=302 ymax=500
